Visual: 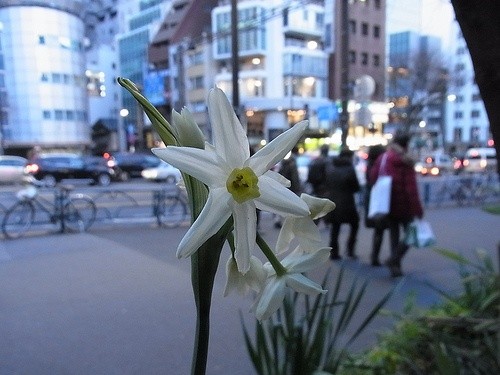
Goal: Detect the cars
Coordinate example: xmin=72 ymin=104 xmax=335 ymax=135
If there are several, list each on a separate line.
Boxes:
xmin=141 ymin=163 xmax=182 ymax=185
xmin=112 ymin=154 xmax=158 ymax=182
xmin=0 ymin=156 xmax=29 ymax=183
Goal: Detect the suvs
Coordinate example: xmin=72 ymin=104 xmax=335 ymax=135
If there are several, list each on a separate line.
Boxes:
xmin=24 ymin=151 xmax=117 ymax=188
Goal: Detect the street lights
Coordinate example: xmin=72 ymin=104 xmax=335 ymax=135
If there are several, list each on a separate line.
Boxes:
xmin=114 ymin=106 xmax=129 ymax=152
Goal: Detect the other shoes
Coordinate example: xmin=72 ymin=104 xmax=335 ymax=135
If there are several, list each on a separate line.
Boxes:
xmin=330 ymin=251 xmax=358 ymax=260
xmin=370 ymin=261 xmax=382 ymax=267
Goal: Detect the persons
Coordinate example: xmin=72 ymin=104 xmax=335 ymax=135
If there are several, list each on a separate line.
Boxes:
xmin=273 ymin=133 xmax=424 ymax=278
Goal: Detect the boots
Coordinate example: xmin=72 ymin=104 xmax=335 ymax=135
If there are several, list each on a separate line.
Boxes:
xmin=384 ymin=241 xmax=409 ymax=277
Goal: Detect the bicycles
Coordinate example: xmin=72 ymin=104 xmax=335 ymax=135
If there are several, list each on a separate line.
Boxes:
xmin=1 ymin=174 xmax=98 ymax=239
xmin=157 ymin=180 xmax=190 ymax=228
xmin=456 ymin=170 xmax=497 ymax=206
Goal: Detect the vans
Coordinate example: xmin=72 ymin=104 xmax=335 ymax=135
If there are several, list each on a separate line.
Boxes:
xmin=422 ymin=154 xmax=453 ymax=176
xmin=464 ymin=147 xmax=498 ymax=172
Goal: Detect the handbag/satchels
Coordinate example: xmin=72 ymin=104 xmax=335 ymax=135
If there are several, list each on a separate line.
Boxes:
xmin=335 ymin=198 xmax=360 ymax=223
xmin=404 ymin=216 xmax=437 ymax=250
xmin=366 ymin=154 xmax=393 ymax=219
